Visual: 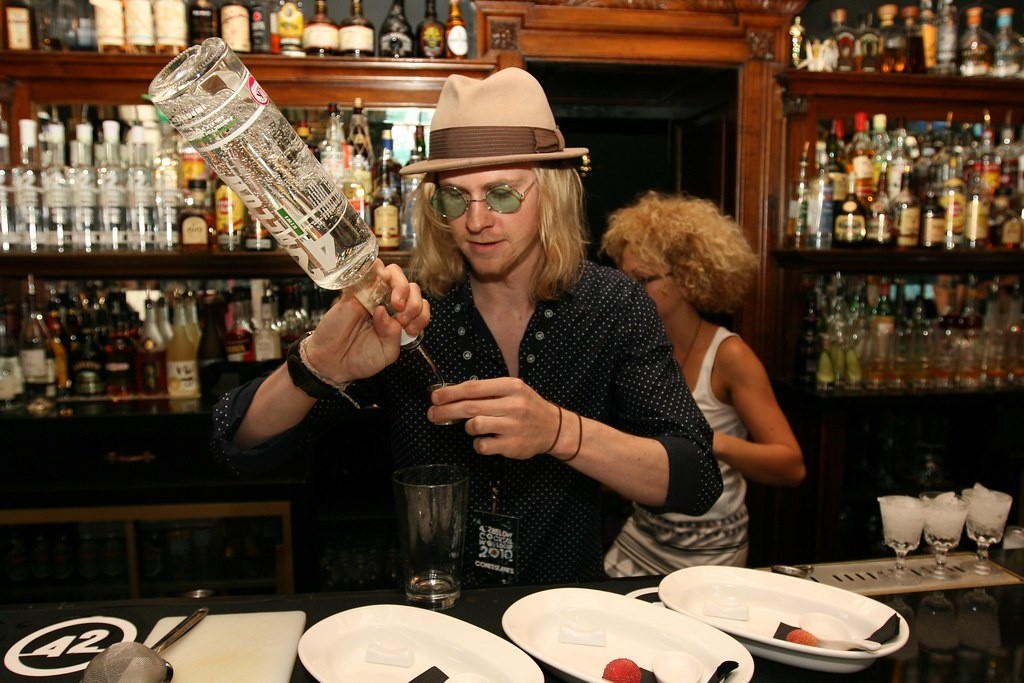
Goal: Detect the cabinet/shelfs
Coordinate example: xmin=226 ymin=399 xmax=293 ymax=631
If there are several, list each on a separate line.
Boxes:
xmin=0 ymin=46 xmax=525 ymax=599
xmin=767 ymin=67 xmax=1023 ymax=561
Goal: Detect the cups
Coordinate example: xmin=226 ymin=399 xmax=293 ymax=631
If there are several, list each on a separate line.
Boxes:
xmin=390 ymin=465 xmax=470 ymax=610
xmin=427 ymin=383 xmax=461 ymax=425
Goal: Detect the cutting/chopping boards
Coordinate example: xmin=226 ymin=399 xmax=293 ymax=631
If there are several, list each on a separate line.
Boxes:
xmin=116 ymin=610 xmax=305 ymax=683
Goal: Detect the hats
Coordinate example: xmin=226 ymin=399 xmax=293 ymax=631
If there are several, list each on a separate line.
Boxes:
xmin=398 ymin=65 xmax=589 ymax=176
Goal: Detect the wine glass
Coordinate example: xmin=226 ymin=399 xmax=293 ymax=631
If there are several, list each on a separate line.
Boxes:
xmin=962 ymin=488 xmax=1013 ymax=577
xmin=919 ymin=491 xmax=962 ymax=579
xmin=877 ymin=495 xmax=929 ymax=584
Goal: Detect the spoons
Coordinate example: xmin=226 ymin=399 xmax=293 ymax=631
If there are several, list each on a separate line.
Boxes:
xmin=444 ymin=673 xmax=491 ymax=683
xmin=652 ymin=651 xmax=703 ymax=683
xmin=799 ymin=612 xmax=881 ymax=651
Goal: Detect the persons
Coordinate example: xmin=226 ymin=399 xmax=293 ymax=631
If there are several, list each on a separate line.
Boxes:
xmin=596 ymin=188 xmax=808 ymax=571
xmin=197 ymin=66 xmax=722 ymax=584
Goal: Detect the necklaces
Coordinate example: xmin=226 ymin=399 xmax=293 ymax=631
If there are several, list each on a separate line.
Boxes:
xmin=679 ymin=318 xmax=706 ymax=369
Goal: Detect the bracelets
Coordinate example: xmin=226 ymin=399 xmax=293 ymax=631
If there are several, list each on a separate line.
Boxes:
xmin=561 ymin=410 xmax=582 ymax=462
xmin=544 ymin=403 xmax=563 ymax=454
xmin=285 ymin=329 xmax=339 ymax=400
xmin=299 ymin=334 xmax=360 ymax=410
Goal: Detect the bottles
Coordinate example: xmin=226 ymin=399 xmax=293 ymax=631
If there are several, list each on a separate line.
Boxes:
xmin=3 ymin=0 xmax=470 ymax=62
xmin=787 ymin=111 xmax=1024 ymax=247
xmin=803 ymin=0 xmax=1024 ymax=77
xmin=0 ymin=97 xmax=428 ymax=407
xmin=147 ymin=36 xmax=425 ymax=350
xmin=790 ymin=270 xmax=1024 ymax=394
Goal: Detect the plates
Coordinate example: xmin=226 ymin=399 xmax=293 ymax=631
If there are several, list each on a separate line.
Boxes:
xmin=658 ymin=565 xmax=910 ymax=673
xmin=502 ymin=588 xmax=754 ymax=683
xmin=297 ymin=604 xmax=544 ymax=683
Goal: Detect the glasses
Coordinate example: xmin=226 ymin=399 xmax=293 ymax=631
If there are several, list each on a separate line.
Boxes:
xmin=632 ymin=269 xmax=681 ymax=289
xmin=430 ymin=175 xmax=536 ymax=219
